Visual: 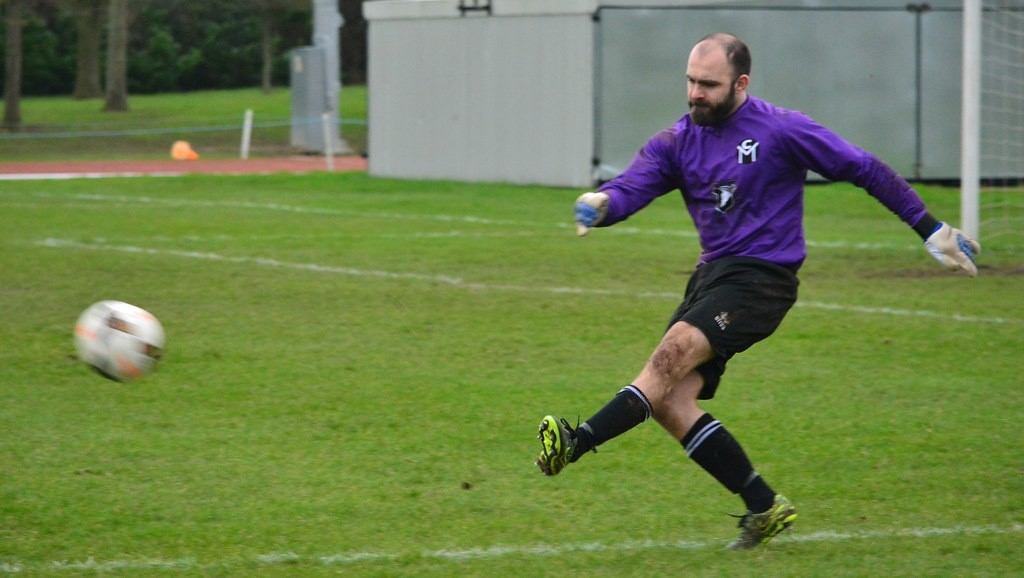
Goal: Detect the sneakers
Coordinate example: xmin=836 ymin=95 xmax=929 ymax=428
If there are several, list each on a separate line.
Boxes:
xmin=535 ymin=415 xmax=581 ymax=477
xmin=724 ymin=495 xmax=796 ymax=552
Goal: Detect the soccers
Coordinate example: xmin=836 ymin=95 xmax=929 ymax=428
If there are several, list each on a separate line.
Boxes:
xmin=73 ymin=299 xmax=167 ymax=385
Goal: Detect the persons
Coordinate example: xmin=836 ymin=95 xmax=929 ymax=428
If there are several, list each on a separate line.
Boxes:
xmin=534 ymin=31 xmax=981 ymax=554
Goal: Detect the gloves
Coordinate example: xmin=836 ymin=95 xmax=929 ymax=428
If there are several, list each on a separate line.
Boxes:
xmin=573 ymin=192 xmax=610 ymax=236
xmin=925 ymin=221 xmax=979 ymax=278
